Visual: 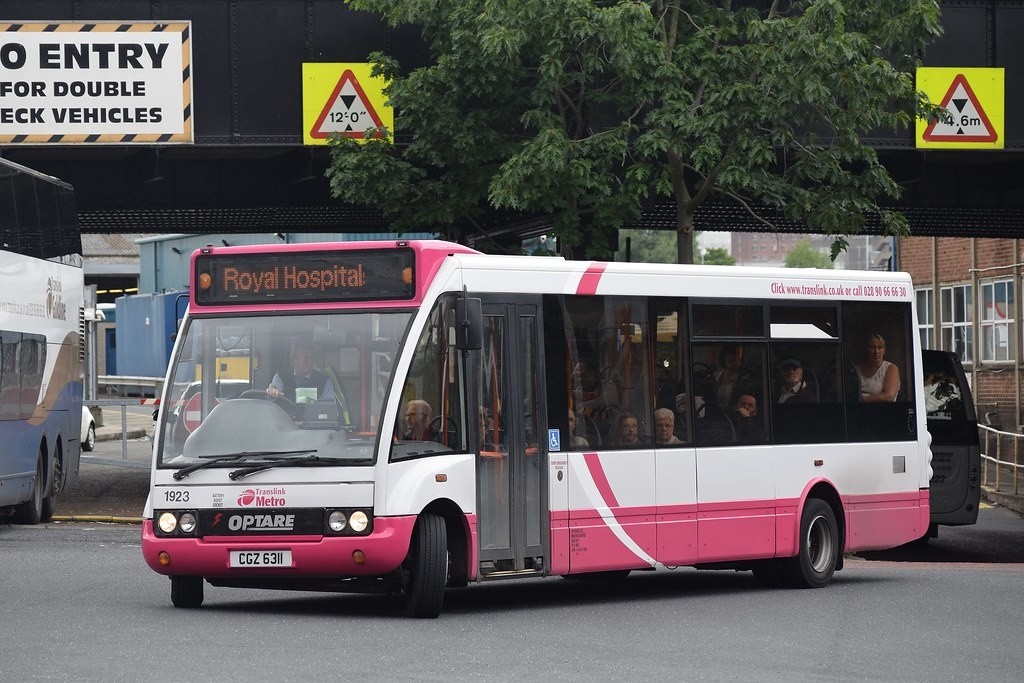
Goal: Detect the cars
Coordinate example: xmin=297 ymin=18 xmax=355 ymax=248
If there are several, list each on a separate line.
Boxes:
xmin=81 ymin=406 xmax=96 ymax=452
xmin=151 ymin=379 xmax=253 ymax=460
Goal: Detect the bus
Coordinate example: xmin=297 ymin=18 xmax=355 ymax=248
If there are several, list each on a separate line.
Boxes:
xmin=139 ymin=240 xmax=933 ymax=619
xmin=0 ymin=158 xmax=84 ymax=526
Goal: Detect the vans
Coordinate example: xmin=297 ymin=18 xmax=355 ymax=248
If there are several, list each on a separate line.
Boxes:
xmin=906 ymin=349 xmax=981 ymax=546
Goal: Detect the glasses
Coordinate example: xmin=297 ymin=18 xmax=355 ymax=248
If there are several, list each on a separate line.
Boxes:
xmin=569 ymin=417 xmax=577 ymax=422
xmin=404 ymin=413 xmax=427 ymax=422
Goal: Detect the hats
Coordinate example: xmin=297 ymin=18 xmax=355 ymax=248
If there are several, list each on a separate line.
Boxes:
xmin=780 ymin=359 xmax=801 ymax=368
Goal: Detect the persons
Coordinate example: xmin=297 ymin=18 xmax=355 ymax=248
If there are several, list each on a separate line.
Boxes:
xmin=569 ymin=344 xmax=822 ymax=451
xmin=479 ymin=405 xmax=492 ymax=451
xmin=263 ymin=341 xmax=340 ymax=418
xmin=851 ymin=333 xmax=902 ymax=403
xmin=402 ymin=399 xmax=442 ymax=443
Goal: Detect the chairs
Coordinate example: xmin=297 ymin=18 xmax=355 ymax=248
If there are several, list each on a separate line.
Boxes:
xmin=573 ymin=358 xmax=861 ymax=450
xmin=427 ymin=415 xmax=459 ymax=448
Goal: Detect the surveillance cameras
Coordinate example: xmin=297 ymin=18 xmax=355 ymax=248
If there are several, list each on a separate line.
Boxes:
xmin=96 ymin=309 xmax=105 ymax=320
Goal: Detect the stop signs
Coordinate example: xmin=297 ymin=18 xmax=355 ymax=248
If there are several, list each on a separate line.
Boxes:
xmin=183 ymin=392 xmax=219 ymax=434
